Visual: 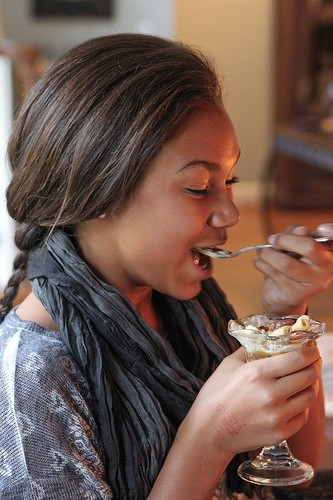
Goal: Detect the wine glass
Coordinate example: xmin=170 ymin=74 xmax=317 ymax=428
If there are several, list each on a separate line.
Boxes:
xmin=225 ymin=312 xmax=327 ymax=487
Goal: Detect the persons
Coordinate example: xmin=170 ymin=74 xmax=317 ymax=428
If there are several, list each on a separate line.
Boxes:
xmin=0 ymin=31 xmax=333 ymax=500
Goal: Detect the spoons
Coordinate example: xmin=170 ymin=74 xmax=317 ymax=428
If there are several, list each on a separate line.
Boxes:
xmin=194 ymin=232 xmax=331 ymax=260
